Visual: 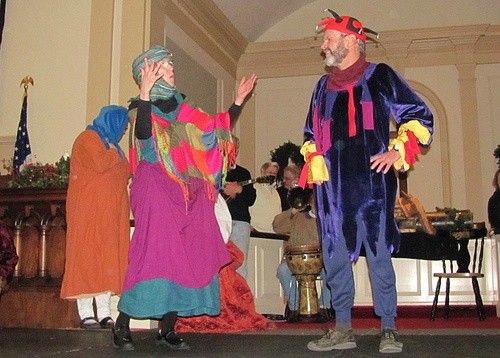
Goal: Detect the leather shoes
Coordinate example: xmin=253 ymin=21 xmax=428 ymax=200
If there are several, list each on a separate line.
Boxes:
xmin=112 ymin=323 xmax=137 ymax=352
xmin=157 ymin=328 xmax=186 ymax=347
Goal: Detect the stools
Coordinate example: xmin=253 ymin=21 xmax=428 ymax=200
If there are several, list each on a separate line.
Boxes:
xmin=285 ymin=273 xmax=331 ymax=322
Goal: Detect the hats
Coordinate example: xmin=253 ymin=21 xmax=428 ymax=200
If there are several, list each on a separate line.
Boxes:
xmin=314 ymin=7 xmax=380 ymax=42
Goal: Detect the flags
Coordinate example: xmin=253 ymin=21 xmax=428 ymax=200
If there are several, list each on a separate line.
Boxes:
xmin=12 ymin=96 xmax=31 ymax=177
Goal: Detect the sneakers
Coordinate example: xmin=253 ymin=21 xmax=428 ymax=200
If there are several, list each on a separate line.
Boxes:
xmin=377 ymin=328 xmax=404 ymax=355
xmin=307 ymin=324 xmax=357 ymax=352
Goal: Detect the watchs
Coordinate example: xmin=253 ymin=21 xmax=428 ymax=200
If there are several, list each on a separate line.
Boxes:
xmin=393 ymin=146 xmax=399 ymax=152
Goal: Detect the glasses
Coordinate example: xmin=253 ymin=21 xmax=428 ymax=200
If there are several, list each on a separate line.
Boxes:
xmin=281 ymin=176 xmax=298 ymax=184
xmin=158 ymin=60 xmax=174 ymax=68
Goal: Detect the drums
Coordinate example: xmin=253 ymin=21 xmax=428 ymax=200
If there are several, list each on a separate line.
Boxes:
xmin=284 ymin=243 xmax=329 ymax=324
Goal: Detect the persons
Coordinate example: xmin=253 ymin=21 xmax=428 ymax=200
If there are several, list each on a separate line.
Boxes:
xmin=302 ymin=7 xmax=433 ymax=355
xmin=112 ymin=45 xmax=334 ymax=350
xmin=485 ymin=169 xmax=500 ymax=237
xmin=60 ymin=105 xmax=132 ymax=330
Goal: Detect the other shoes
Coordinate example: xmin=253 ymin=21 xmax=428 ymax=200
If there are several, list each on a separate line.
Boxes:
xmin=100 ymin=316 xmax=114 ymax=329
xmin=79 ymin=317 xmax=102 ymax=329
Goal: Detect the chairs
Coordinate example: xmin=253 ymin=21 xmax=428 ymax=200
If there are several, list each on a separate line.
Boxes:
xmin=430 ymin=221 xmax=487 ymax=321
